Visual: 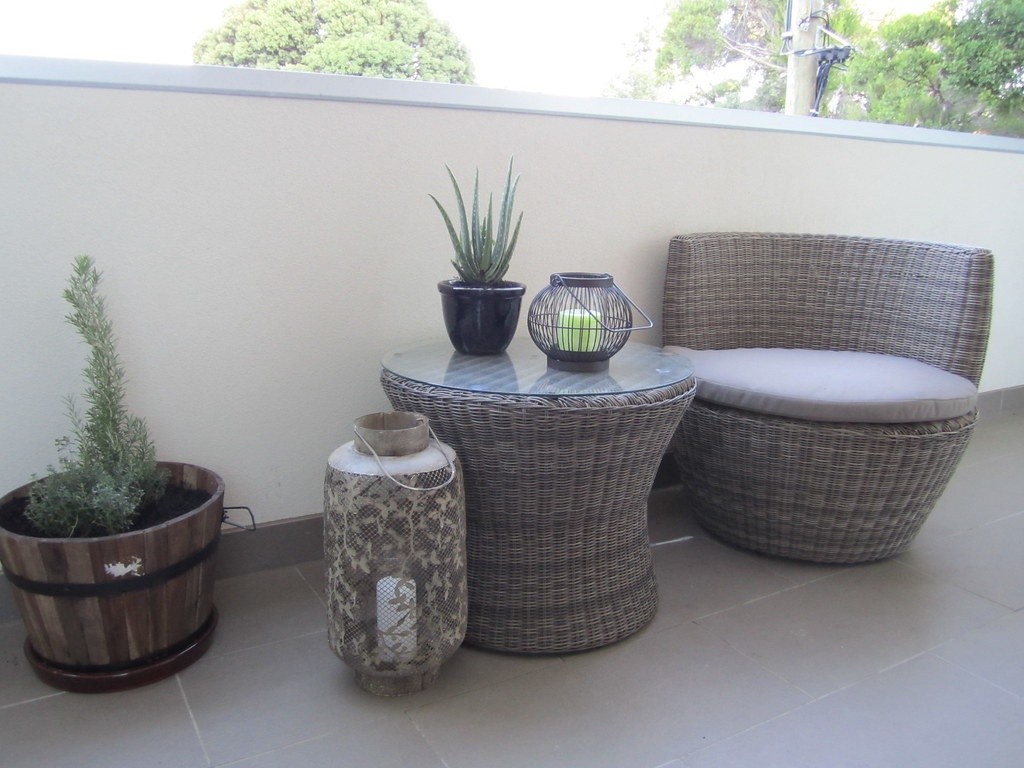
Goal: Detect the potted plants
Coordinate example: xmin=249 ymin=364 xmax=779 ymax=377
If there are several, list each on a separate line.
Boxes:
xmin=0 ymin=254 xmax=227 ymax=698
xmin=428 ymin=152 xmax=526 ymax=357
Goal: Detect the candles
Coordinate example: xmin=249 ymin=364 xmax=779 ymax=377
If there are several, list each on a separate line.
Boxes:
xmin=560 ymin=309 xmax=602 ymax=352
xmin=376 ymin=576 xmax=417 ymax=663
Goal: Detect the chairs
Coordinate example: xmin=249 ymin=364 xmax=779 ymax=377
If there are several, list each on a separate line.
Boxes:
xmin=662 ymin=229 xmax=993 ymax=566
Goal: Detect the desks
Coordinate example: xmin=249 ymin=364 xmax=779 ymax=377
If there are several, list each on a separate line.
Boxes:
xmin=376 ymin=338 xmax=698 ymax=657
